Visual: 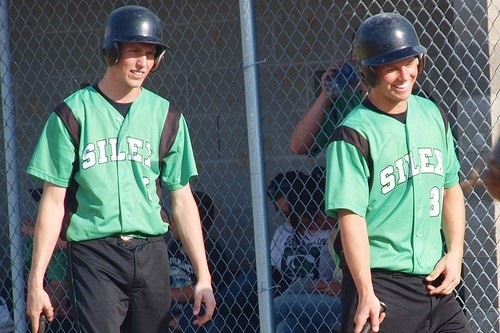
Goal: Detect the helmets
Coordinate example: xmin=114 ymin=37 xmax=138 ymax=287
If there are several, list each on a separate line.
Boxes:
xmin=354 ymin=13 xmax=427 ymax=87
xmin=101 ymin=5 xmax=169 ymax=72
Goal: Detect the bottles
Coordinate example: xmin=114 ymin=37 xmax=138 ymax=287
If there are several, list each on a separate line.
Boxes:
xmin=324 ymin=60 xmax=359 ymax=96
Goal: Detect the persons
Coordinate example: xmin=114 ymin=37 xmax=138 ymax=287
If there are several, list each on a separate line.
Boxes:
xmin=325 ymin=12 xmax=474 ymax=333
xmin=288 ymin=24 xmax=372 ymax=157
xmin=26 ymin=5 xmax=217 ymax=333
xmin=20 ymin=167 xmax=344 ymax=333
